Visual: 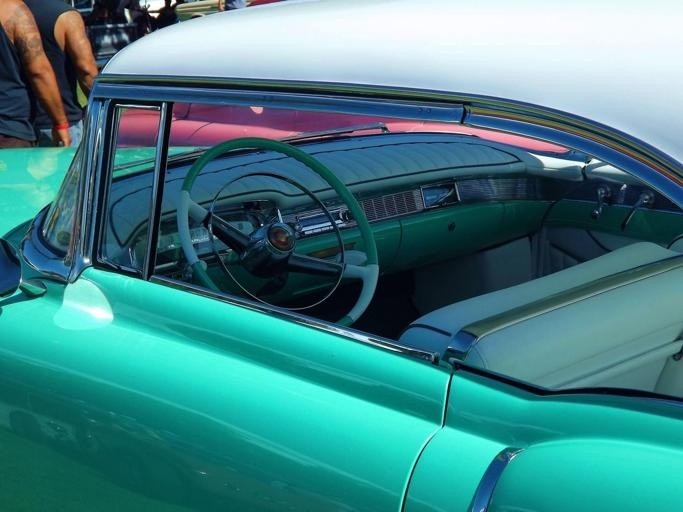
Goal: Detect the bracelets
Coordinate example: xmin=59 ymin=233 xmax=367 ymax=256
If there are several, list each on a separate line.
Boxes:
xmin=52 ymin=122 xmax=69 ymax=130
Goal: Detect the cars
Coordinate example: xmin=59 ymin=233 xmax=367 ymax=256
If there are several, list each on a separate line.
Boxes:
xmin=0 ymin=0 xmax=681 ymax=511
xmin=75 ymin=1 xmax=225 ymax=68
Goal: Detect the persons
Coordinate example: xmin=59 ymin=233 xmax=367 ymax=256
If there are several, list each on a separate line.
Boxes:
xmin=0 ymin=0 xmax=70 ymax=147
xmin=156 ymin=1 xmax=173 ymax=26
xmin=22 ymin=1 xmax=98 ymax=147
xmin=217 ymin=1 xmax=251 ymax=12
